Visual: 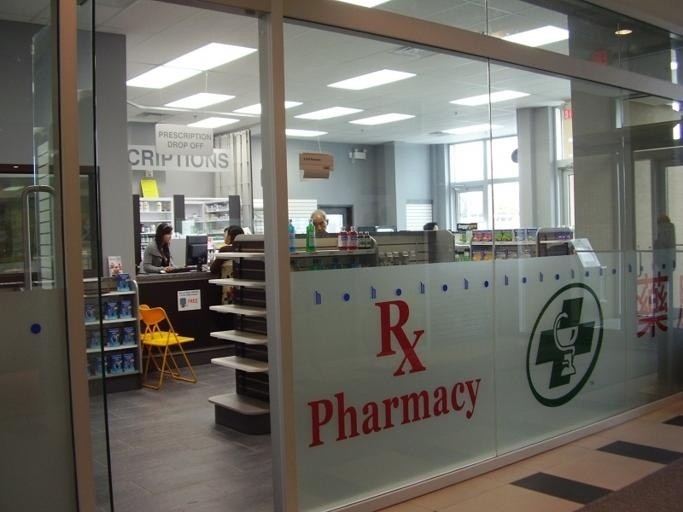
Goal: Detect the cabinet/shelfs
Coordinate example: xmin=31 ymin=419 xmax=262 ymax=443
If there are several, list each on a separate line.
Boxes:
xmin=452 ymin=222 xmax=571 ymax=263
xmin=174 ymin=194 xmax=232 ymax=270
xmin=133 ymin=196 xmax=174 ymax=270
xmin=207 ymin=253 xmax=272 ymax=439
xmin=82 ymin=281 xmax=142 ymax=399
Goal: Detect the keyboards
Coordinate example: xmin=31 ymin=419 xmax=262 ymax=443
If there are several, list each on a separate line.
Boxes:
xmin=165 ymin=267 xmax=197 ymax=273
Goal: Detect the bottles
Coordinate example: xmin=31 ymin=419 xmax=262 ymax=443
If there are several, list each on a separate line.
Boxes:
xmin=305 ymin=218 xmax=316 ymax=253
xmin=288 ymin=219 xmax=295 ymax=252
xmin=338 ymin=226 xmax=369 ymax=249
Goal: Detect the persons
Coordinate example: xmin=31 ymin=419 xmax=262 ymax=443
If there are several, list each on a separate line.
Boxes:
xmin=312 ymin=209 xmax=329 ymax=234
xmin=209 ymin=224 xmax=244 ymax=279
xmin=423 ymin=221 xmax=440 ymax=231
xmin=142 ymin=223 xmax=175 ymax=275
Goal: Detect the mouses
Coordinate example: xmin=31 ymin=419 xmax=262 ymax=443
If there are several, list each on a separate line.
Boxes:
xmin=159 ymin=270 xmax=166 ymax=274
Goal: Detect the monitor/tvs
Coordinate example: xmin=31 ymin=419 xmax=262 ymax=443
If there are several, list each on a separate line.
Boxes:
xmin=185 ymin=234 xmax=207 ymax=272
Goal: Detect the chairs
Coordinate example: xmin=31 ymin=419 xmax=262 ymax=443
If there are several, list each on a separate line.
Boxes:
xmin=138 ymin=305 xmax=197 ymax=389
xmin=138 ymin=302 xmax=181 ymax=378
